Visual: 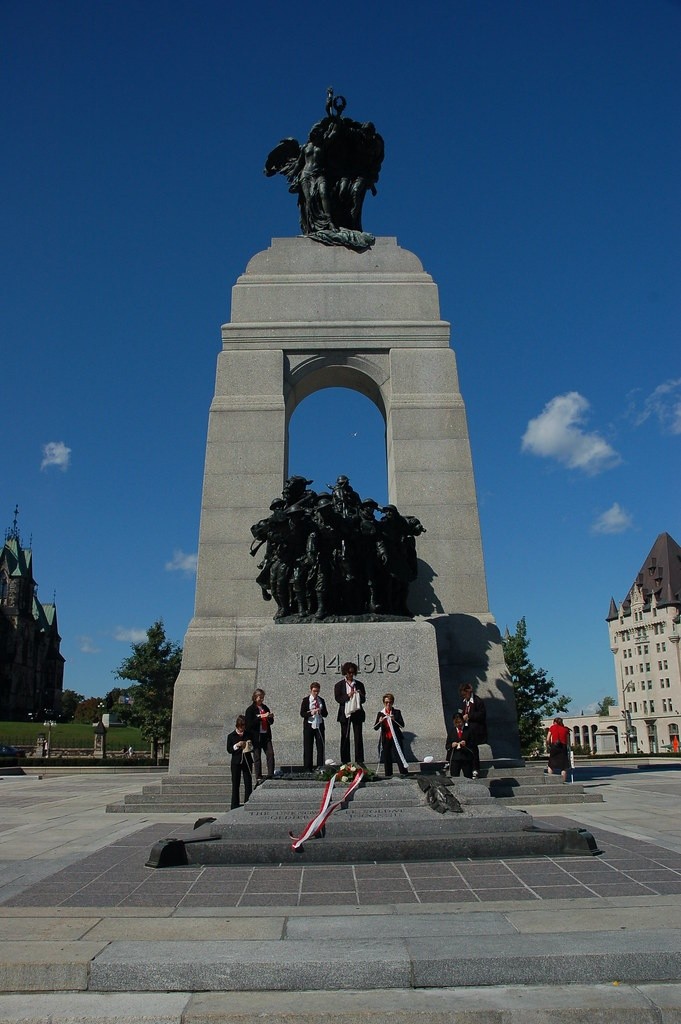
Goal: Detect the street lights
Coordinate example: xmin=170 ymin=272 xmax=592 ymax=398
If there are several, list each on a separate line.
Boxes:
xmin=623 ymin=680 xmax=635 ymax=753
xmin=44 ymin=720 xmax=56 ymax=758
xmin=98 ymin=702 xmax=105 ymax=724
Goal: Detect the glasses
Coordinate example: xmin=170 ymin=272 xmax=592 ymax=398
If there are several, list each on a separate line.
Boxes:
xmin=348 ymin=671 xmax=355 ymax=675
xmin=384 ymin=701 xmax=392 ymax=704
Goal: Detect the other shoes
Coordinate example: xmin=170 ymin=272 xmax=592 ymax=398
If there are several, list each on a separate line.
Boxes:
xmin=357 ymin=762 xmax=364 ymax=765
xmin=340 ymin=762 xmax=347 ymax=765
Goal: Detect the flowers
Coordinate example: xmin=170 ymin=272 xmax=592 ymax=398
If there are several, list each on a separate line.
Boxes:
xmin=336 ymin=764 xmax=358 ymax=782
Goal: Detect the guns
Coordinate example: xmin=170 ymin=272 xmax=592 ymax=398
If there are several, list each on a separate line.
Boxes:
xmin=252 ymin=498 xmax=426 ymax=534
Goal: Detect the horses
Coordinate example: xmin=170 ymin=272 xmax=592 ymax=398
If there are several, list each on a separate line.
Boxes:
xmin=326 ymin=478 xmax=352 ymax=518
xmin=281 ymin=475 xmax=313 ymax=506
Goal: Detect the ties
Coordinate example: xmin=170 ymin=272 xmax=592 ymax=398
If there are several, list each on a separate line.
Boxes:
xmin=457 ymin=727 xmax=462 ymax=739
xmin=260 ymin=709 xmax=267 ymax=731
xmin=314 ymin=697 xmax=319 ymax=710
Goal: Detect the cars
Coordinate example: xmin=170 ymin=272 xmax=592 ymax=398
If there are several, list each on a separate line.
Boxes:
xmin=0 ymin=745 xmax=26 ymax=758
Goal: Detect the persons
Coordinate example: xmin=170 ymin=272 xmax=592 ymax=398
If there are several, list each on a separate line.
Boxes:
xmin=299 ymin=682 xmax=328 ymax=765
xmin=122 ymin=745 xmax=127 ymax=755
xmin=458 ymin=684 xmax=486 ymax=746
xmin=251 ymin=475 xmax=423 ymax=618
xmin=334 ymin=662 xmax=366 ymax=763
xmin=227 ymin=715 xmax=252 ymax=809
xmin=546 ymin=717 xmax=569 ymax=781
xmin=243 ymin=689 xmax=279 ymax=781
xmin=129 ymin=744 xmax=133 ymax=756
xmin=374 ymin=694 xmax=409 ymax=779
xmin=446 ymin=713 xmax=480 ymax=779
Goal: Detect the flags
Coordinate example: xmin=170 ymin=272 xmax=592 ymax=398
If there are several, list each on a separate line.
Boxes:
xmin=674 ymin=736 xmax=678 ymax=752
